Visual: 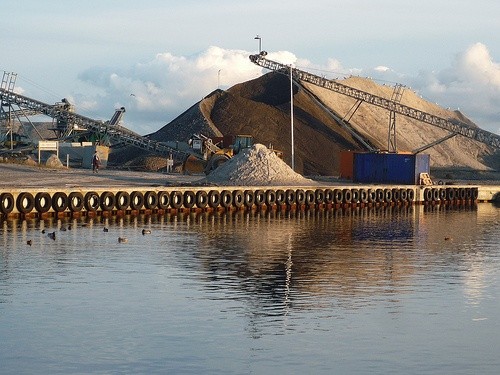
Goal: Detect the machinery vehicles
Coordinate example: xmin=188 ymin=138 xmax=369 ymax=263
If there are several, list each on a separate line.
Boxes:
xmin=185 ymin=134 xmax=283 ymax=178
xmin=5 ymin=132 xmax=29 ymax=148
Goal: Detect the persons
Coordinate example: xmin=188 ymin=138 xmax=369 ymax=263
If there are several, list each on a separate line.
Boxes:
xmin=90 ymin=151 xmax=101 ymax=174
